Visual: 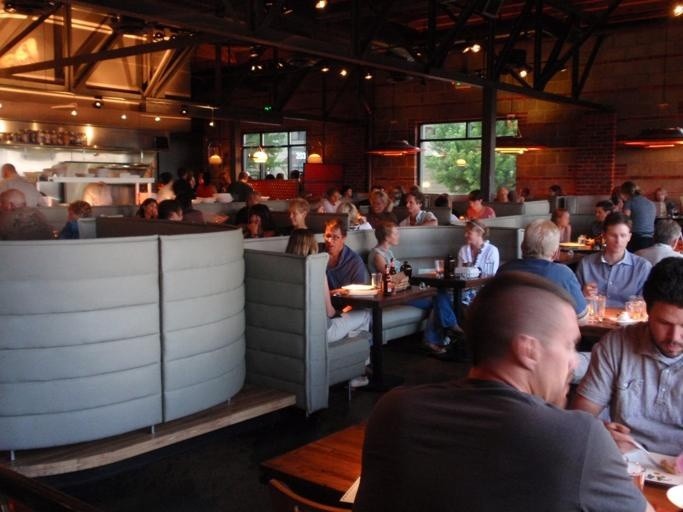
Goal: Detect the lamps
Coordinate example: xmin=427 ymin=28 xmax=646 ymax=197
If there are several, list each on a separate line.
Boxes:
xmin=251 ymin=129 xmax=268 ymax=164
xmin=365 ymin=116 xmax=421 ymax=158
xmin=307 ymin=126 xmax=323 ymax=164
xmin=207 ymin=138 xmax=225 ymax=165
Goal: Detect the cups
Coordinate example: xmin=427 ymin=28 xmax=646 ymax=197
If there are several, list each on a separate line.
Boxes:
xmin=594 ymin=295 xmax=606 ymax=319
xmin=371 ymin=272 xmax=382 ymax=289
xmin=628 ymin=475 xmax=644 ymax=492
xmin=487 ymin=261 xmax=494 ymax=276
xmin=630 ymin=295 xmax=645 ymax=318
xmin=625 ymin=302 xmax=639 ymax=319
xmin=435 ymin=260 xmax=444 ymax=278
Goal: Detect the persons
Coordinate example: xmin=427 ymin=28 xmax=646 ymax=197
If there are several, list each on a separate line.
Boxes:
xmin=495 ymin=219 xmax=589 ymax=328
xmin=496 ymin=187 xmax=508 ymax=203
xmin=366 ymin=220 xmax=464 ymax=357
xmin=548 ymin=185 xmax=560 ymax=196
xmin=589 ymin=200 xmax=614 ymax=239
xmin=454 ymin=218 xmax=499 ymax=305
xmin=620 ymin=181 xmax=657 ymax=249
xmin=136 ymin=198 xmax=159 ymax=222
xmin=248 ymin=204 xmax=278 ymax=239
xmin=228 ymin=171 xmax=253 ymax=200
xmin=388 ymin=189 xmax=401 ymax=213
xmin=434 ymin=193 xmax=459 ymax=224
xmin=655 ymin=187 xmax=678 ymax=218
xmin=520 ymin=188 xmax=530 ymax=202
xmin=285 ymin=228 xmax=372 ymax=389
xmin=173 ymin=166 xmax=192 ymax=199
xmin=368 ymin=191 xmax=394 ymax=227
xmin=340 ymin=184 xmax=355 ymax=202
xmin=287 ymin=196 xmax=310 ymax=231
xmin=59 ymin=199 xmax=92 ymax=239
xmin=195 ymin=171 xmax=217 ymax=197
xmin=574 ymin=211 xmax=653 ymax=309
xmin=636 ymin=218 xmax=683 ymax=266
xmin=321 ymin=188 xmax=341 ymax=213
xmin=235 ymin=190 xmax=260 ymax=224
xmin=319 ymin=216 xmax=369 ymax=293
xmin=465 ymin=189 xmax=495 ymax=220
xmin=0 ymin=188 xmax=58 ymax=241
xmin=570 ymin=256 xmax=683 ymax=465
xmin=551 ymin=208 xmax=571 ymax=250
xmin=507 ymin=191 xmax=518 ymax=203
xmin=336 ymin=202 xmax=372 ymax=231
xmin=291 ymin=169 xmax=302 ymax=194
xmin=0 ymin=161 xmax=40 ymax=209
xmin=399 ymin=191 xmax=439 ymax=227
xmin=159 ymin=200 xmax=183 ymax=223
xmin=349 ymin=272 xmax=658 ymax=512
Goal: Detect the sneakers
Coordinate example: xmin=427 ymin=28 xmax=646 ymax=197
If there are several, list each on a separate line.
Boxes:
xmin=446 ymin=328 xmax=467 ymax=339
xmin=350 ymin=375 xmax=370 ymax=388
xmin=422 ymin=337 xmax=448 ymax=355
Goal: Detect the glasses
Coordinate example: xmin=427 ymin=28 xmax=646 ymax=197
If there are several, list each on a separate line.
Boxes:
xmin=323 ymin=233 xmax=346 ymax=242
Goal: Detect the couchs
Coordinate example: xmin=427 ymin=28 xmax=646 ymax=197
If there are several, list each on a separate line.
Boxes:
xmin=479 ymin=209 xmax=608 ymax=244
xmin=1 ymin=214 xmax=246 ymax=454
xmin=248 ymin=225 xmax=524 ymax=416
xmin=553 ymin=194 xmax=619 ymax=230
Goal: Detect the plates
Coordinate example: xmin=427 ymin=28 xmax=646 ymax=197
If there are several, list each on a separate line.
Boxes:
xmin=622 ymin=450 xmax=683 ymax=486
xmin=341 ymin=283 xmax=372 ymax=291
xmin=666 ymin=484 xmax=683 ymax=510
xmin=558 ymin=242 xmax=586 ymax=248
xmin=610 ymin=318 xmax=648 ymax=326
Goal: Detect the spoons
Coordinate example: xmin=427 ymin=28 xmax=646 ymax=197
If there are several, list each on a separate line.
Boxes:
xmin=621 ymin=432 xmax=675 ymax=474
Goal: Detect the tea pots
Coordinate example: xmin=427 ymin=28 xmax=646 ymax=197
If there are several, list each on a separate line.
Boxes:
xmin=445 ymin=254 xmax=457 ymax=278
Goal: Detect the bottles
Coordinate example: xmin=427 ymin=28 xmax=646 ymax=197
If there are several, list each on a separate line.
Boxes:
xmin=16 ymin=128 xmax=87 ymax=147
xmin=443 ymin=254 xmax=456 ymax=278
xmin=382 ymin=265 xmax=392 ymax=296
xmin=389 ymin=259 xmax=396 ymax=275
